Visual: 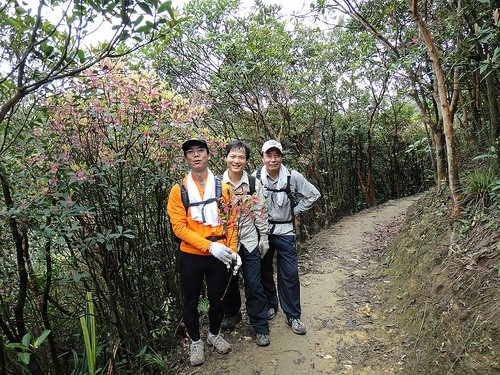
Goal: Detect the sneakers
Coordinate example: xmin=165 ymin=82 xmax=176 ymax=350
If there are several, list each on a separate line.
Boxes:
xmin=256 ymin=334 xmax=270 ymax=345
xmin=268 ymin=308 xmax=274 ymax=320
xmin=206 ymin=329 xmax=232 ymax=354
xmin=190 ymin=340 xmax=205 ymax=366
xmin=221 ymin=308 xmax=242 ymax=329
xmin=285 ymin=316 xmax=306 ymax=334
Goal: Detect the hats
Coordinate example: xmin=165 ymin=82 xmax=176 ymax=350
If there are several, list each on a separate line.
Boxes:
xmin=261 ymin=140 xmax=283 ymax=154
xmin=182 ymin=138 xmax=207 ymax=146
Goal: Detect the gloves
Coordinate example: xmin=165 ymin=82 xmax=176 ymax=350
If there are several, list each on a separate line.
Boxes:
xmin=208 ymin=242 xmax=232 ymax=265
xmin=227 ymin=253 xmax=242 ymax=276
xmin=259 ymin=235 xmax=269 ymax=258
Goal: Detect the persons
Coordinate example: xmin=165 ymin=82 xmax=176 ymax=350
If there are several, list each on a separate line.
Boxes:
xmin=167 ymin=139 xmax=322 ymax=364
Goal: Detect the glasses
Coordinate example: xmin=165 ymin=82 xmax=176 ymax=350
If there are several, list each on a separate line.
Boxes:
xmin=185 ymin=148 xmax=207 ymax=154
